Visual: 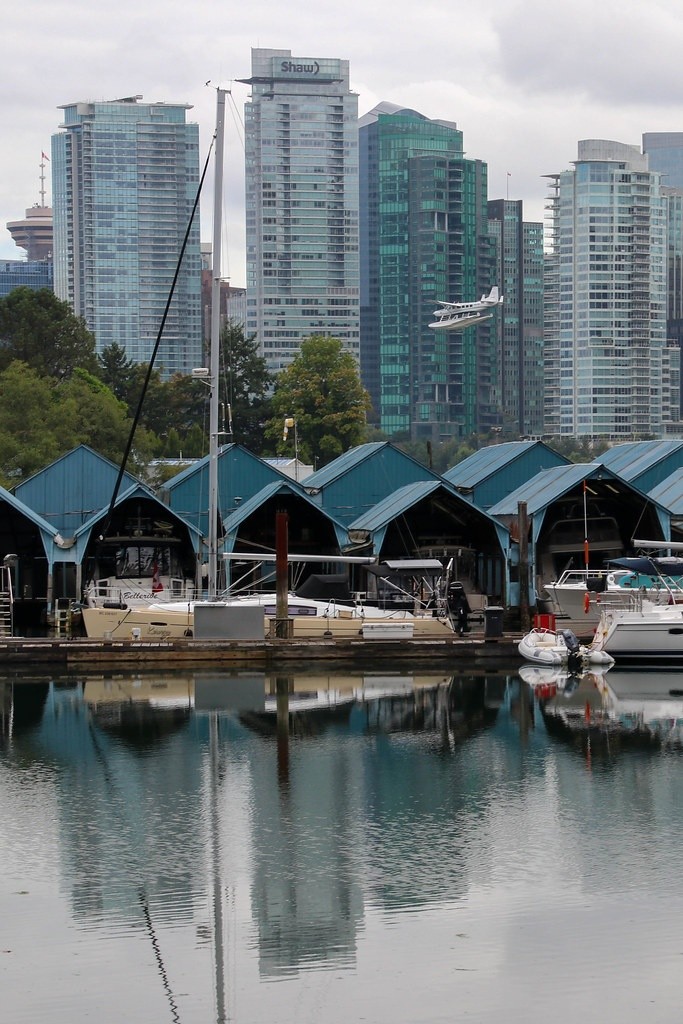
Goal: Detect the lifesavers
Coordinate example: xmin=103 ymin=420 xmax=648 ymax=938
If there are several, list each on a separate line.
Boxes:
xmin=583 ymin=593 xmax=590 ymax=614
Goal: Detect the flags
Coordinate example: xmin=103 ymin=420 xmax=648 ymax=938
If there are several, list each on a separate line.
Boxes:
xmin=152 ymin=557 xmax=164 ymax=593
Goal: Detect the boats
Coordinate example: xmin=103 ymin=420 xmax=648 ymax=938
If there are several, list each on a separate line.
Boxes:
xmin=541 ymin=482 xmax=683 ymax=622
xmin=589 ymin=538 xmax=683 ymax=663
xmin=518 ymin=625 xmax=614 ymax=666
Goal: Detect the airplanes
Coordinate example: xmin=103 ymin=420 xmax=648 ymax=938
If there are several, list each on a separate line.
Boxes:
xmin=427 ymin=286 xmax=504 ymax=331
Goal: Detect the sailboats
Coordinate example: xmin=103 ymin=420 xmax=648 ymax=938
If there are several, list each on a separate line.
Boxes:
xmin=73 ymin=86 xmax=461 ymax=640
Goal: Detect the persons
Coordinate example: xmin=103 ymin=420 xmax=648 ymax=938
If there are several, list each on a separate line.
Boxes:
xmin=451 ymin=581 xmax=474 ymax=637
xmin=201 ymin=560 xmax=209 ymax=589
xmin=171 ymin=557 xmax=186 ymax=593
